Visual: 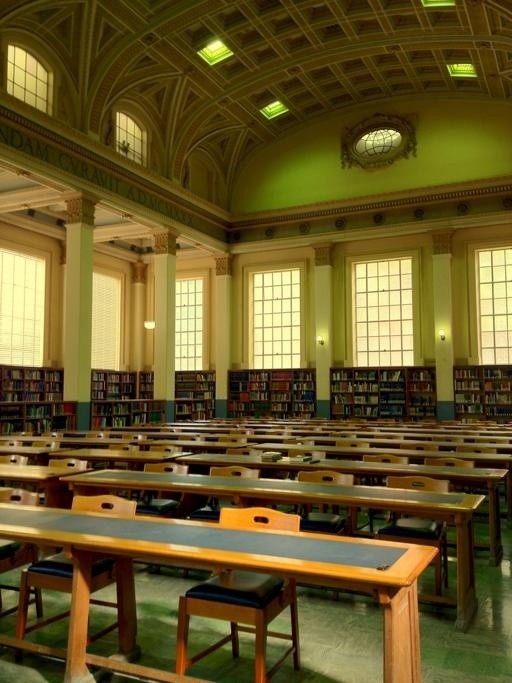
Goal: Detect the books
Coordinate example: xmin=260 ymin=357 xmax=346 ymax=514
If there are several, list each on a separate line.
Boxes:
xmin=0 ymin=367 xmax=77 ymax=434
xmin=175 ymin=372 xmax=215 ymax=421
xmin=91 ymin=370 xmax=154 ymax=429
xmin=330 ymin=370 xmax=435 ymax=419
xmin=275 ymin=458 xmax=321 ymax=465
xmin=282 ymin=455 xmax=311 ymax=462
xmin=452 ymin=367 xmax=511 ymax=423
xmin=261 ymin=450 xmax=281 ymax=462
xmin=228 ymin=370 xmax=315 ymax=418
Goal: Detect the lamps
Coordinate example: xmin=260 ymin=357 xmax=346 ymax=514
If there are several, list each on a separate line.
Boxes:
xmin=144 ymin=320 xmax=155 ymax=336
xmin=438 ymin=330 xmax=446 ymax=340
xmin=316 ymin=335 xmax=324 ymax=346
